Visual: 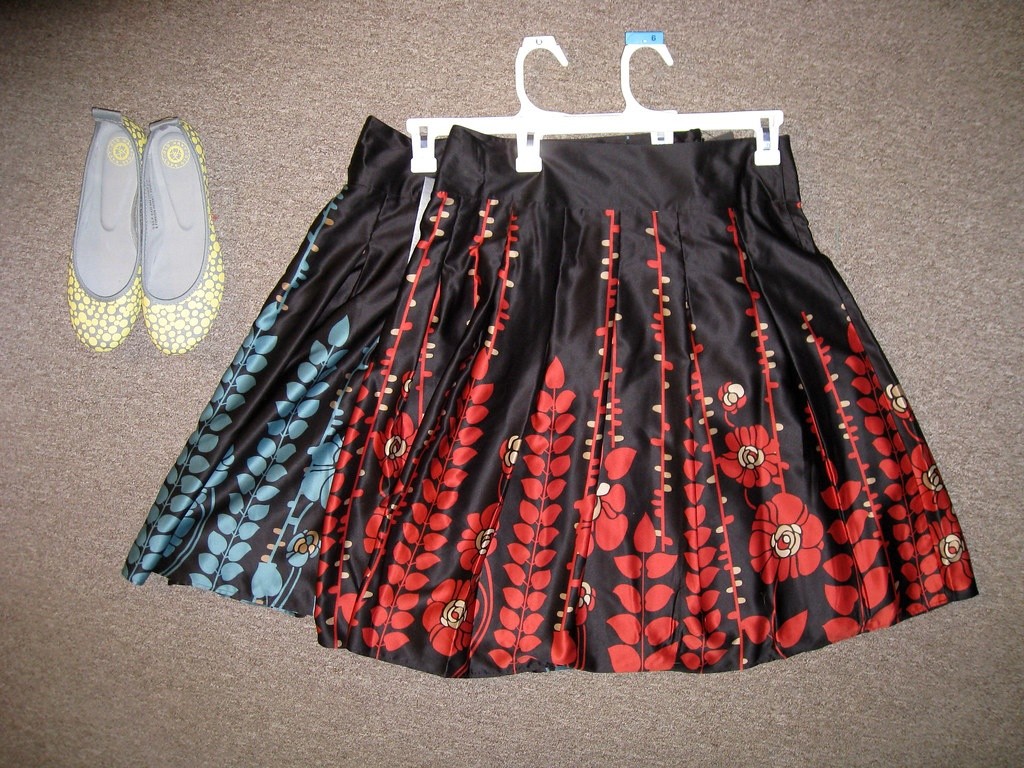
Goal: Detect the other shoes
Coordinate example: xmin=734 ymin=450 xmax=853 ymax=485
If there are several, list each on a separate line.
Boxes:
xmin=140 ymin=116 xmax=225 ymax=354
xmin=68 ymin=106 xmax=143 ymax=352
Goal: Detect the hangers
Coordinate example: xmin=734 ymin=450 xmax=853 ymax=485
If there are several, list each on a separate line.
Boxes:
xmin=515 ymin=31 xmax=784 ymax=173
xmin=406 ymin=36 xmax=679 ymax=172
